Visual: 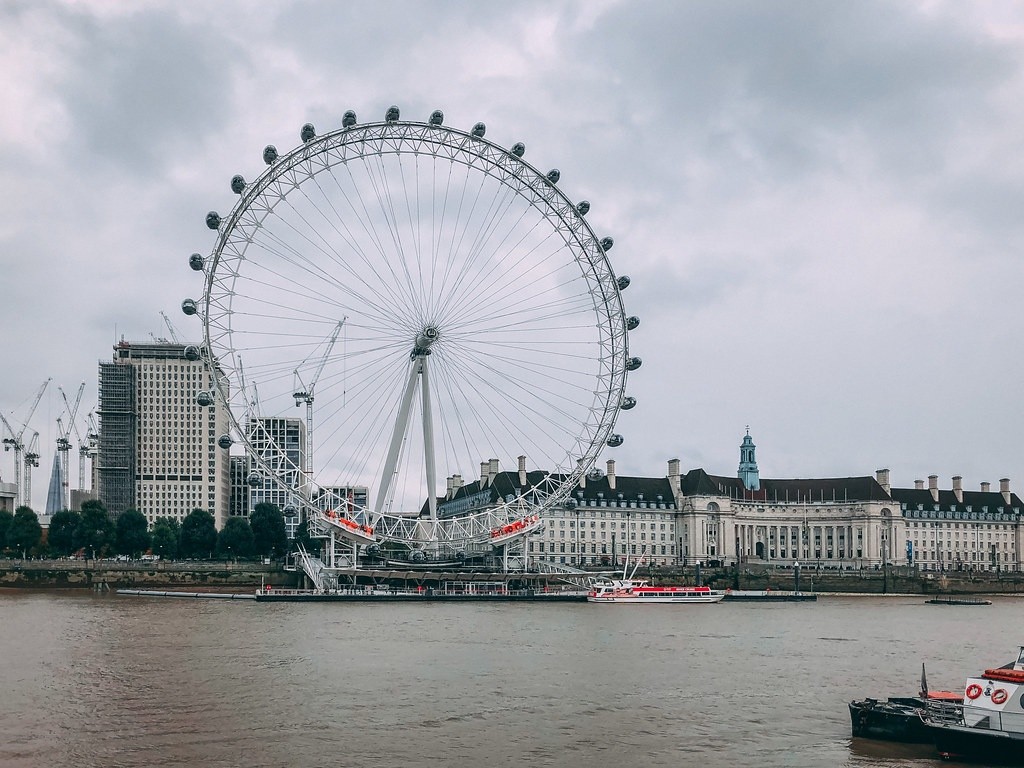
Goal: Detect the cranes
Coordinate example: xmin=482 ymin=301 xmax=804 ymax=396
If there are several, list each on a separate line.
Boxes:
xmin=293 ymin=316 xmax=347 ymax=482
xmin=160 ymin=311 xmax=185 ymax=343
xmin=253 ymin=381 xmax=261 ymax=417
xmin=59 ymin=383 xmax=85 ymax=506
xmin=22 ymin=423 xmax=40 ymax=507
xmin=0 ymin=378 xmax=53 ymax=509
xmin=237 ymin=355 xmax=249 ymax=414
xmin=57 ymin=387 xmax=98 ymax=490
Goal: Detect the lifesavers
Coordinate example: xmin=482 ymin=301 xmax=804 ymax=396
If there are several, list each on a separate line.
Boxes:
xmin=991 ymin=689 xmax=1008 ymax=704
xmin=965 ymin=684 xmax=982 ymax=699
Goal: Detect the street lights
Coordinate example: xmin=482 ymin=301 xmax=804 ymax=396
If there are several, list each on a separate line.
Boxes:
xmin=611 ymin=535 xmax=615 ymax=554
xmin=627 ymin=511 xmax=631 ymax=554
xmin=794 ymin=563 xmax=799 ymax=591
xmin=160 ymin=545 xmax=163 ymax=561
xmin=576 ymin=511 xmax=580 ymax=564
xmin=741 ymin=547 xmax=742 ymax=563
xmin=272 ymin=545 xmax=275 ymax=561
xmin=696 ymin=560 xmax=700 ymax=586
xmin=817 ymin=557 xmax=820 ymax=569
xmin=228 ymin=546 xmax=231 ymax=560
xmin=840 ymin=557 xmax=843 ymax=569
xmin=89 ymin=544 xmax=92 ymax=559
xmin=860 ymin=558 xmax=863 ymax=569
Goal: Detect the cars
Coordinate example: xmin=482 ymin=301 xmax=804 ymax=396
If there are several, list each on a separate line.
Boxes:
xmin=72 ymin=554 xmax=83 ymax=560
xmin=29 ymin=553 xmax=47 ymax=561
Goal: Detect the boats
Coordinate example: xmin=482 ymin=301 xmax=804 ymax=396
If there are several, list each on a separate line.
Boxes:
xmin=915 ymin=645 xmax=1024 ymax=762
xmin=923 ymin=599 xmax=992 ymax=605
xmin=587 ymin=550 xmax=724 ymax=603
